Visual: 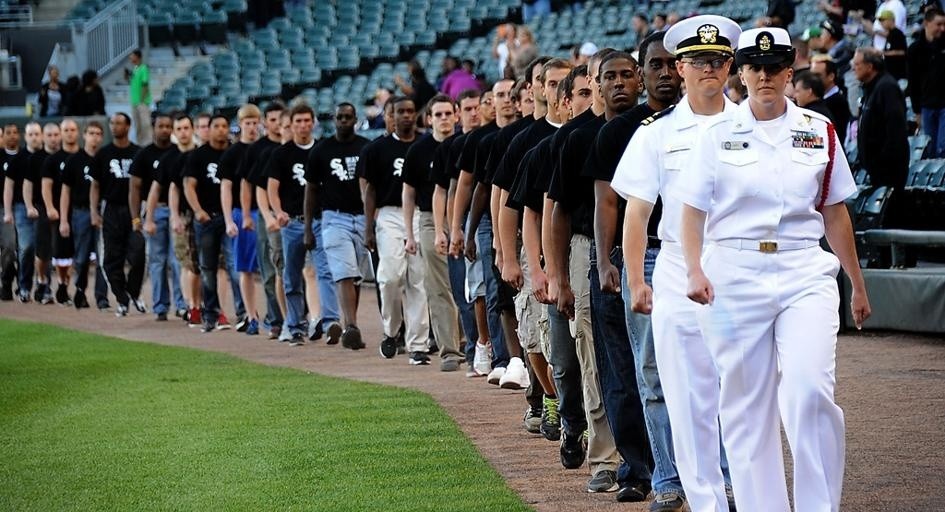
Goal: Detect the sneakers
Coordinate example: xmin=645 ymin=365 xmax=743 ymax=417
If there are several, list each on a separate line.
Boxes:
xmin=587 ymin=470 xmax=619 ymax=493
xmin=380 ymin=337 xmax=397 ymax=358
xmin=499 ymin=356 xmax=531 ymax=390
xmin=559 ymin=422 xmax=587 ymax=470
xmin=157 ymin=306 xmax=324 ymax=347
xmin=523 ymin=404 xmax=545 ymax=432
xmin=342 ymin=325 xmax=363 ymax=351
xmin=616 ymin=480 xmax=652 ymax=502
xmin=325 ymin=322 xmax=343 ymax=346
xmin=407 ymin=351 xmax=431 ymax=365
xmin=650 ymin=492 xmax=685 ymax=512
xmin=440 ymin=361 xmax=459 ymax=372
xmin=473 ymin=341 xmax=492 ymax=377
xmin=541 ymin=392 xmax=562 ymax=442
xmin=2 ymin=286 xmax=148 ymax=318
xmin=488 ymin=365 xmax=507 ymax=386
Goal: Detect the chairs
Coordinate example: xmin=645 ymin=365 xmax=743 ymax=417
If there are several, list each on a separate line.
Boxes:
xmin=62 ymin=1 xmax=822 ymax=128
xmin=823 ymin=1 xmax=945 ymax=268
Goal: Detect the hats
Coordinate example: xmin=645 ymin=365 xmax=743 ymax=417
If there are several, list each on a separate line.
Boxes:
xmin=798 ymin=26 xmax=822 ymax=42
xmin=875 ymin=10 xmax=896 ymax=20
xmin=662 ymin=13 xmax=743 ymax=61
xmin=578 ymin=42 xmax=600 ymax=57
xmin=737 ymin=28 xmax=795 ymax=63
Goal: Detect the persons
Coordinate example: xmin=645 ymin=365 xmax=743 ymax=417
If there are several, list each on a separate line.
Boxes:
xmin=0 ymin=0 xmax=945 ymax=512
